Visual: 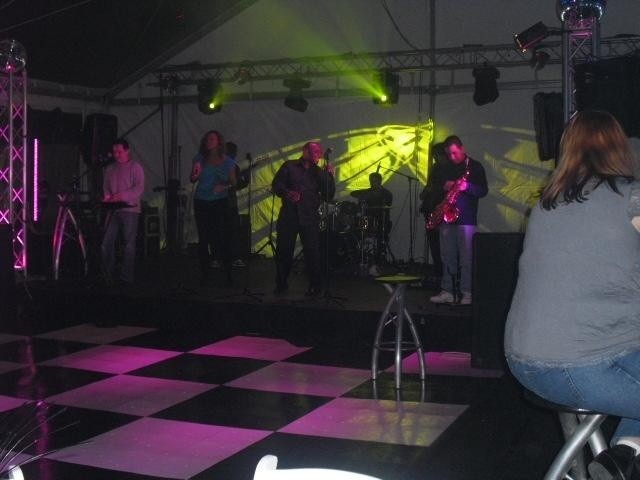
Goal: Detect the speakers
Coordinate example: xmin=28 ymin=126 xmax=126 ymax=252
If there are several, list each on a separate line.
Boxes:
xmin=575 ymin=52 xmax=640 ymax=129
xmin=472 ymin=232 xmax=525 ymax=370
xmin=534 ymin=94 xmax=563 ymax=163
xmin=239 ymin=215 xmax=251 ymax=257
xmin=85 ymin=115 xmax=116 ymax=166
xmin=1 ymin=224 xmax=18 ymax=335
xmin=137 ymin=207 xmax=159 ymax=272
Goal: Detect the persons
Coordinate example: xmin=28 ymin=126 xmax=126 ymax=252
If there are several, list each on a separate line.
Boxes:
xmin=270 ymin=142 xmax=336 ymax=301
xmin=190 ymin=130 xmax=237 ymax=290
xmin=351 ymin=172 xmax=393 ymax=279
xmin=429 ymin=134 xmax=488 ymax=305
xmin=95 ymin=138 xmax=145 ymax=290
xmin=420 ymin=142 xmax=451 ymax=288
xmin=503 ymin=109 xmax=640 ymax=479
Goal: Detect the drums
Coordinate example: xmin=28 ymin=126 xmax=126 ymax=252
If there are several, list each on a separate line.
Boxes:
xmin=368 ymin=205 xmax=393 ymax=231
xmin=319 ymin=228 xmax=361 ymax=279
xmin=338 ymin=201 xmax=359 ymax=233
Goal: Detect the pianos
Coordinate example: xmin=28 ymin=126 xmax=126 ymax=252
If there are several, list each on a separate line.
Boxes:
xmin=62 ymin=200 xmax=137 ymax=209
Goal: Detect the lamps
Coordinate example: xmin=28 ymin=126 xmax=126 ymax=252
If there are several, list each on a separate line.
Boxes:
xmin=197 ymin=82 xmax=225 ymax=116
xmin=511 ymin=19 xmax=563 ymax=54
xmin=368 ymin=65 xmax=401 ymax=107
xmin=281 ymin=75 xmax=312 ymax=113
xmin=470 ymin=61 xmax=503 ymax=107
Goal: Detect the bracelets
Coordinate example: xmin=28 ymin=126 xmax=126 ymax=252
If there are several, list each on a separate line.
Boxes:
xmin=222 ymin=182 xmax=233 ymax=192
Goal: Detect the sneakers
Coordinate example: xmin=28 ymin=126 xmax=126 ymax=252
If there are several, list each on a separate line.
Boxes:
xmin=431 ymin=291 xmax=472 ymax=305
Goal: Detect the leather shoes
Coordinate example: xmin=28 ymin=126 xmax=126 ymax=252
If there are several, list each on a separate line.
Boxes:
xmin=587 ymin=444 xmax=640 ymax=480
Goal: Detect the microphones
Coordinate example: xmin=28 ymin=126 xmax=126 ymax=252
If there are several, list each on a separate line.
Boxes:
xmin=325 ymin=144 xmax=336 ymax=155
xmin=102 ymin=151 xmax=113 ymax=166
xmin=375 ymin=164 xmax=380 ymax=176
xmin=194 ymin=154 xmax=201 ymax=173
xmin=244 ymin=153 xmax=253 ymax=163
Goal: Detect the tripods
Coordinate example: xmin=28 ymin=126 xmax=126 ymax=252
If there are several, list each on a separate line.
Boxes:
xmin=252 ymin=195 xmax=277 ymax=258
xmin=303 ymin=156 xmax=348 ymax=308
xmin=397 ymin=173 xmax=419 ymax=275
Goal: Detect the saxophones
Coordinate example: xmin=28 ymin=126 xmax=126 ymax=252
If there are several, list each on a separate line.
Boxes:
xmin=426 ymin=169 xmax=469 ymax=229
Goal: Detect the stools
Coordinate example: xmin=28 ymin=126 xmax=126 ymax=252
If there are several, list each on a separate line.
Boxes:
xmin=539 ymin=404 xmax=613 ymax=480
xmin=372 ymin=274 xmax=432 ymax=391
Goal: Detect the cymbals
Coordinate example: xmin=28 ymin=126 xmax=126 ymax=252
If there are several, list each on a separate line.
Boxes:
xmin=352 ymin=190 xmax=382 ymax=200
